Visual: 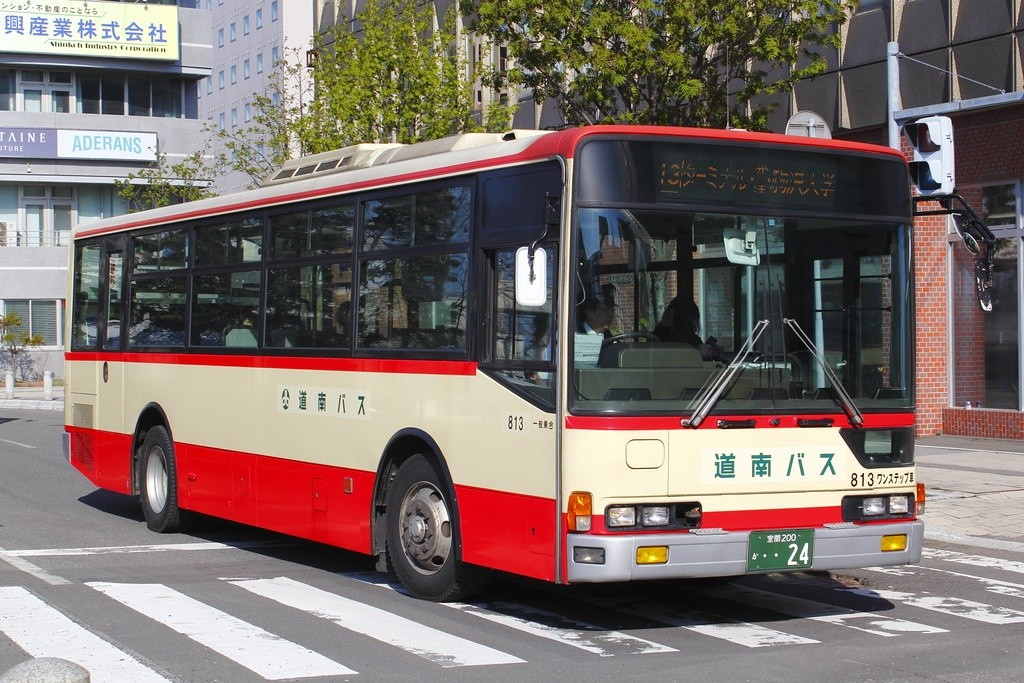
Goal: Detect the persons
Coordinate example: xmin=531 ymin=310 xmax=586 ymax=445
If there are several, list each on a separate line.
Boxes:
xmin=536 ymin=282 xmax=616 ymax=379
xmin=235 ymin=300 xmax=367 ymax=349
xmin=649 ymin=298 xmax=725 ymax=363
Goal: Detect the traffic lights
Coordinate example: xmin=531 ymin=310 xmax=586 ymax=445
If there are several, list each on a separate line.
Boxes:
xmin=908 ymin=115 xmax=956 ymax=194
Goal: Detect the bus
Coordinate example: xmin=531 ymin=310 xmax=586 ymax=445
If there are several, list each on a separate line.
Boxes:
xmin=63 ymin=124 xmax=995 ymax=603
